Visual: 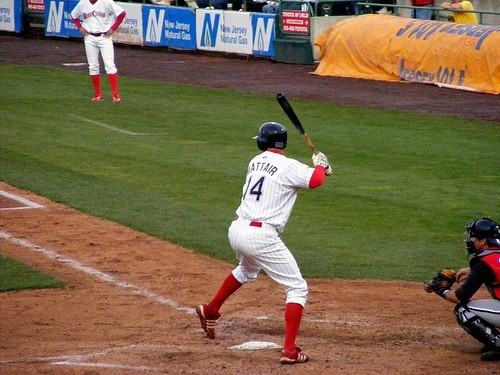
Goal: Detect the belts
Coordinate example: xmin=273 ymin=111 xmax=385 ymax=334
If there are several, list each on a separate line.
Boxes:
xmin=89 ymin=32 xmax=108 ymax=38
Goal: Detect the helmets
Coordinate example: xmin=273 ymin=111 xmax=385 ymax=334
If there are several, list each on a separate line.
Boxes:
xmin=464 ymin=216 xmax=500 ymax=239
xmin=252 ymin=122 xmax=288 ymax=149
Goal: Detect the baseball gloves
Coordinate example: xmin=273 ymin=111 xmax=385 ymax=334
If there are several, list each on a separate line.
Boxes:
xmin=424 ymin=268 xmax=457 ymax=294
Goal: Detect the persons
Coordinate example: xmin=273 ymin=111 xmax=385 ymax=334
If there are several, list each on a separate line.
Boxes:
xmin=431 ymin=217 xmax=500 ymax=361
xmin=71 ymin=0 xmax=127 ymax=103
xmin=409 ymin=0 xmax=435 ymax=20
xmin=149 ymin=0 xmax=399 ymax=15
xmin=196 ymin=121 xmax=331 ymax=364
xmin=440 ymin=0 xmax=478 ymax=25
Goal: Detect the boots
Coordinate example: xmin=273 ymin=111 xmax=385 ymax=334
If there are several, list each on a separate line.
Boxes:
xmin=459 ymin=316 xmax=500 ymax=361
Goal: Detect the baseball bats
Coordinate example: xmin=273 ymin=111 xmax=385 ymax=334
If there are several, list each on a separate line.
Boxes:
xmin=275 ymin=92 xmax=318 ymax=155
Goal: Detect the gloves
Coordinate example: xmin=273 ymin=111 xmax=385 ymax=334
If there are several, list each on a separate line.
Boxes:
xmin=312 ymin=151 xmax=333 ymax=176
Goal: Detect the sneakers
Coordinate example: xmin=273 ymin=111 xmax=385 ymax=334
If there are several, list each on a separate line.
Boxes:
xmin=280 ymin=346 xmax=309 ymax=365
xmin=90 ymin=94 xmax=104 ymax=102
xmin=112 ymin=94 xmax=121 ymax=103
xmin=195 ymin=303 xmax=222 ymax=340
